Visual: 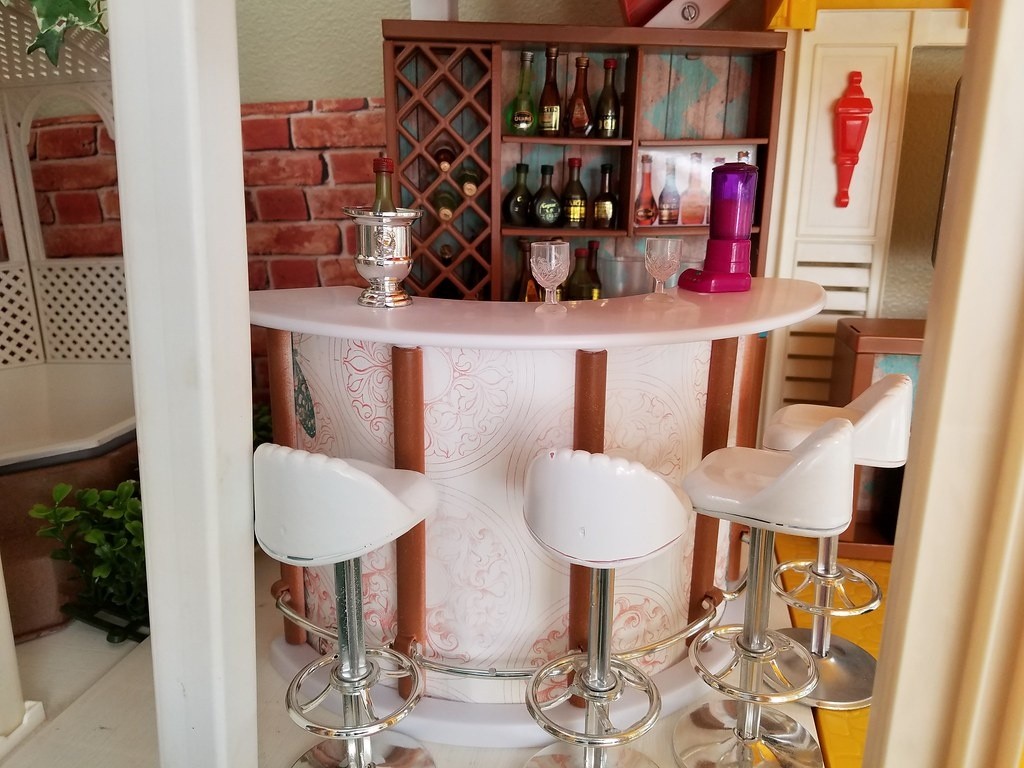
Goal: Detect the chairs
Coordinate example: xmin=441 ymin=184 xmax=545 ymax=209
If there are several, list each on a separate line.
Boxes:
xmin=681 ymin=417 xmax=855 ymax=768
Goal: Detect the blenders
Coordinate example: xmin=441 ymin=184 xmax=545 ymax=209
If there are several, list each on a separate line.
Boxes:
xmin=677 ymin=163 xmax=758 ymax=293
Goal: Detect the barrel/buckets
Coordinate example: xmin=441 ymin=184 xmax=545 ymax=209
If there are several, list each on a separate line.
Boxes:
xmin=341 ymin=206 xmax=424 ymax=307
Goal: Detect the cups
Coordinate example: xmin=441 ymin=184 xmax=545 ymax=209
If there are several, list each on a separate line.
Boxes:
xmin=596 ymin=255 xmax=646 ymax=299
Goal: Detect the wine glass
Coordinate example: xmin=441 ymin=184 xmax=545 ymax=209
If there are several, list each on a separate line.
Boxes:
xmin=645 ymin=238 xmax=683 ymax=293
xmin=531 ymin=243 xmax=567 ymax=316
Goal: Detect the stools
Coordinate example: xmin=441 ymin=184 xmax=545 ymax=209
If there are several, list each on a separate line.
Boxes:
xmin=522 ymin=446 xmax=690 ymax=768
xmin=253 ymin=442 xmax=437 ymax=768
xmin=762 ymin=373 xmax=912 ymax=710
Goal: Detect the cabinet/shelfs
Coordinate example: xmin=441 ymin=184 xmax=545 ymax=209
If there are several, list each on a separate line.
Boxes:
xmin=493 ymin=38 xmax=785 ymax=237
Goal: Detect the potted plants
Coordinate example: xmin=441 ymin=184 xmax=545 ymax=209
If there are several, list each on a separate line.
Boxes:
xmin=27 ymin=403 xmax=272 ymax=646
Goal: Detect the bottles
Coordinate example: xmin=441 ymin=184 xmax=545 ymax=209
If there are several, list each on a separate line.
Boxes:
xmin=587 ymin=241 xmax=602 ymax=300
xmin=562 ymin=57 xmax=594 ymax=137
xmin=538 ymin=47 xmax=561 ymax=137
xmin=567 ymin=249 xmax=592 ymax=300
xmin=456 ymin=169 xmax=480 ymax=196
xmin=510 ymin=237 xmax=563 ymax=302
xmin=504 ymin=50 xmax=539 ymax=136
xmin=436 ymin=190 xmax=456 ymax=220
xmin=634 ymin=155 xmax=658 ymax=225
xmin=527 ymin=165 xmax=563 ymax=228
xmin=371 ymin=158 xmax=397 ymax=214
xmin=659 ymin=158 xmax=680 ymax=224
xmin=560 ymin=158 xmax=587 ymax=228
xmin=593 ymin=59 xmax=620 ymax=138
xmin=592 ymin=164 xmax=617 ymax=229
xmin=707 ymin=151 xmax=750 ymax=164
xmin=440 ymin=244 xmax=453 ymax=267
xmin=680 ymin=152 xmax=707 ymax=224
xmin=503 ymin=163 xmax=533 ymax=226
xmin=434 ymin=140 xmax=456 ymax=171
